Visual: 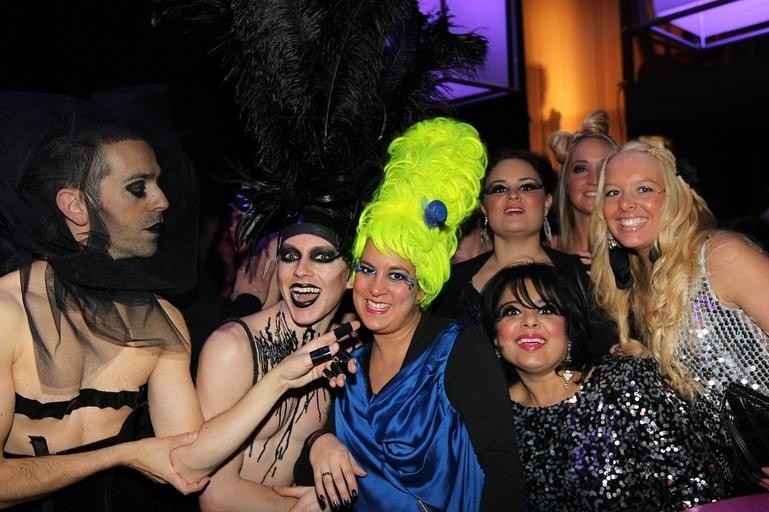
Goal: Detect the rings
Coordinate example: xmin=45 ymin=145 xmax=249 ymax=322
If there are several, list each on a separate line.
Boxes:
xmin=323 ymin=472 xmax=331 ymax=476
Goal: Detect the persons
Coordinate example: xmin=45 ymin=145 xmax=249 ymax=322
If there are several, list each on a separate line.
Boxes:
xmin=292 ymin=224 xmax=528 ymax=511
xmin=423 ymin=110 xmax=769 ymax=511
xmin=2 ymin=111 xmax=362 ymax=511
xmin=156 ymin=191 xmax=371 ymax=511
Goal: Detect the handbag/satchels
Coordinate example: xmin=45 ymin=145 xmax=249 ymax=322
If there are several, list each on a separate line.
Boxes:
xmin=720 ymin=383 xmax=769 ymax=495
